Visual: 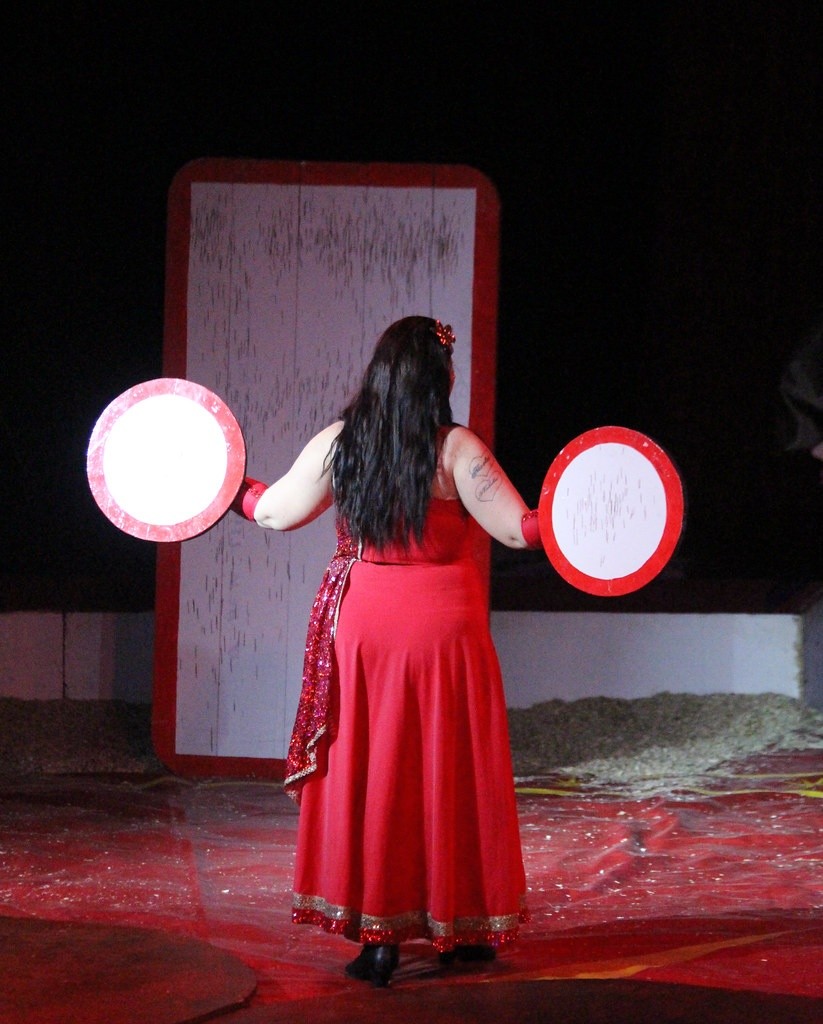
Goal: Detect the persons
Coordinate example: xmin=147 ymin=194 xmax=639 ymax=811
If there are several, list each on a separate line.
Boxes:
xmin=230 ymin=316 xmax=544 ymax=988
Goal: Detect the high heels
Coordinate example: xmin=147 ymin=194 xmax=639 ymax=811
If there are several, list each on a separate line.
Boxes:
xmin=345 ymin=943 xmax=398 ymax=986
xmin=439 ymin=943 xmax=496 ymax=965
